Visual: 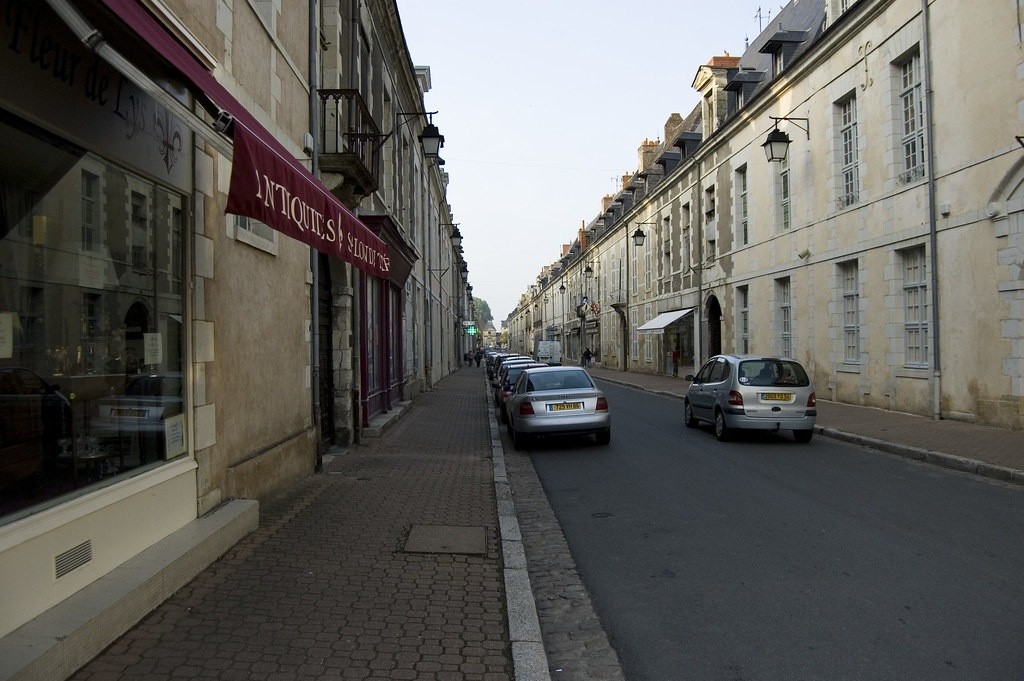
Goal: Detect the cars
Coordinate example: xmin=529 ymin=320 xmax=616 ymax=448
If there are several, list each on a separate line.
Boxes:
xmin=684 ymin=352 xmax=818 ymax=446
xmin=91 ymin=370 xmax=186 ymax=448
xmin=0 ymin=365 xmax=77 ymax=449
xmin=502 ymin=365 xmax=611 ymax=447
xmin=485 ymin=345 xmax=548 ymax=417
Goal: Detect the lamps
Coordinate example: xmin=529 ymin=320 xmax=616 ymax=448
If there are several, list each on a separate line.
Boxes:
xmin=584 ymin=261 xmax=600 ymax=278
xmin=396 ymin=110 xmax=445 ymax=159
xmin=439 ymin=222 xmax=464 ymax=247
xmin=631 ymin=222 xmax=657 ymax=246
xmin=558 ymin=274 xmax=570 ymax=293
xmin=515 ymin=294 xmax=553 ymax=322
xmin=453 ymin=261 xmax=474 ymax=303
xmin=760 ymin=115 xmax=810 ymax=163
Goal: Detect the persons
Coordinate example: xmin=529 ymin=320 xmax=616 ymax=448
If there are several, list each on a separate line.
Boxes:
xmin=474 ymin=351 xmax=483 ymax=368
xmin=583 ymin=348 xmax=594 ymax=369
xmin=467 ymin=351 xmax=473 ymax=367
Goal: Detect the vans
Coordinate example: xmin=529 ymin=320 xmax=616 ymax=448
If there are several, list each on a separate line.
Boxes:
xmin=530 ymin=341 xmax=561 ymax=365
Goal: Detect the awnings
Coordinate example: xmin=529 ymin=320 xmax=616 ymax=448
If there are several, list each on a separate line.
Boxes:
xmin=47 ymin=0 xmax=391 ymax=282
xmin=636 ymin=307 xmax=695 ymax=335
